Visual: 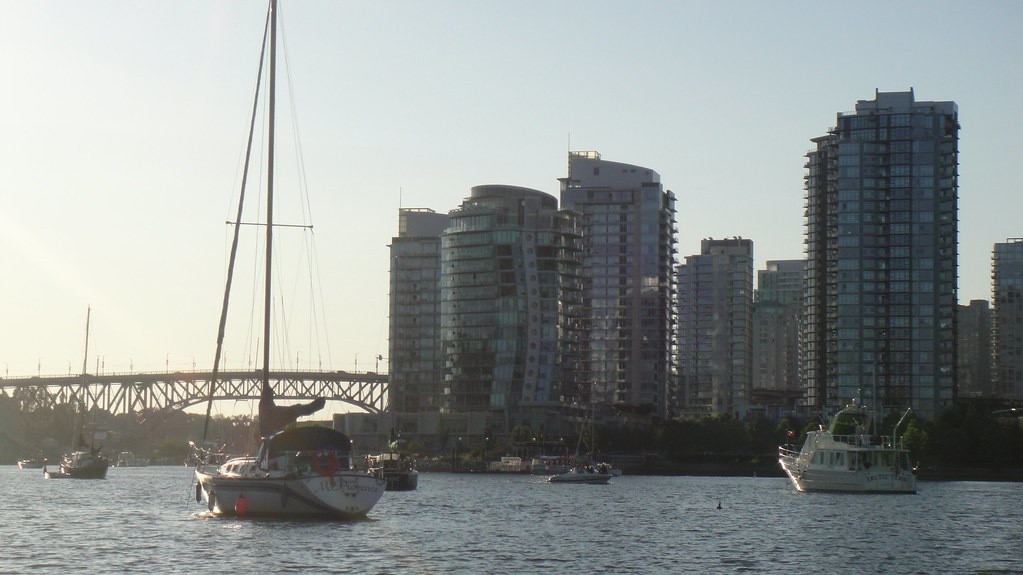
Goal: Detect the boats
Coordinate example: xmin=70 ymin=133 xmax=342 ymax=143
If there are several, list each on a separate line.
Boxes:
xmin=110 ymin=451 xmax=151 ymax=467
xmin=779 ymin=388 xmax=919 ymax=495
xmin=365 ymin=427 xmax=418 ymax=491
xmin=17 ymin=448 xmax=47 ymax=469
xmin=184 ymin=455 xmax=197 ymax=467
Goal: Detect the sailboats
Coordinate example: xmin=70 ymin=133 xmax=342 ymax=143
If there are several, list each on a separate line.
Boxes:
xmin=195 ymin=0 xmax=389 ymax=520
xmin=44 ymin=307 xmax=110 ymax=480
xmin=548 ymin=381 xmax=613 ymax=483
